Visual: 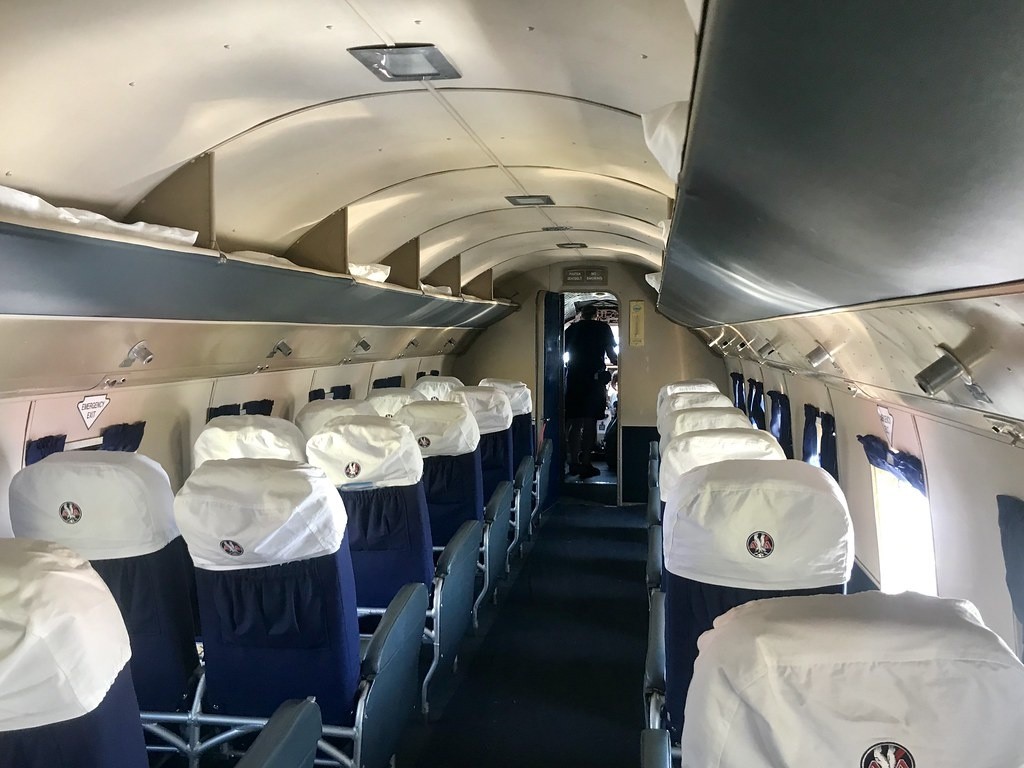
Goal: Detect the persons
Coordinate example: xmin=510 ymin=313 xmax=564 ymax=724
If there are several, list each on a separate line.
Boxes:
xmin=565 ymin=305 xmax=617 ymax=477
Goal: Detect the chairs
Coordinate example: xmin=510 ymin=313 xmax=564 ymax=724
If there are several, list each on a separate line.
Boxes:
xmin=656 ymin=378 xmax=720 ymax=416
xmin=193 ymin=415 xmax=307 ymax=469
xmin=392 ymin=400 xmax=514 ymax=627
xmin=0 ymin=537 xmax=322 ymax=768
xmin=365 ymin=388 xmax=429 ymax=418
xmin=306 ymin=415 xmax=483 ymax=720
xmin=411 ymin=376 xmax=464 ymax=400
xmin=295 ymin=400 xmax=379 ymax=442
xmin=444 ymin=386 xmax=535 ymax=576
xmin=645 ymin=428 xmax=786 ymax=596
xmin=643 ymin=460 xmax=855 ymax=758
xmin=478 ymin=377 xmax=556 ymax=540
xmin=647 ymin=407 xmax=752 ymax=488
xmin=640 ymin=591 xmax=1024 ymax=768
xmin=649 ymin=392 xmax=734 ymax=457
xmin=9 ymin=450 xmax=207 ymax=744
xmin=172 ymin=458 xmax=428 ymax=768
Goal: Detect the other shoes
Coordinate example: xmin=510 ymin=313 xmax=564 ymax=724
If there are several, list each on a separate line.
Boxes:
xmin=568 ymin=462 xmax=580 ymax=476
xmin=579 ymin=462 xmax=601 ymax=479
